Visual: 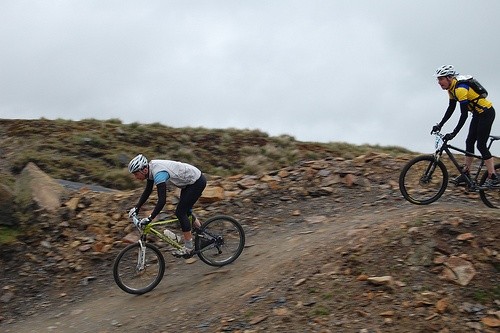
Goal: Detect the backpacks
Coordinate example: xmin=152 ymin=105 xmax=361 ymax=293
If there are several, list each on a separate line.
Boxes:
xmin=453 ymin=78 xmax=488 ymax=102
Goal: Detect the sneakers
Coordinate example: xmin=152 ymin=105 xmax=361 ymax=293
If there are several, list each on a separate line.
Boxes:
xmin=447 ymin=175 xmax=467 ymax=186
xmin=479 ymin=177 xmax=500 ymax=190
xmin=171 ymin=245 xmax=195 ymax=257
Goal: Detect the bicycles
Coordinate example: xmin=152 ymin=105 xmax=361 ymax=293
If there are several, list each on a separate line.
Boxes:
xmin=398 ymin=128 xmax=500 ymax=209
xmin=112 ymin=209 xmax=246 ymax=296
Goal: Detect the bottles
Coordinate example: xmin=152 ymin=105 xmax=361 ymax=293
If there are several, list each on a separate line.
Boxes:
xmin=162 ymin=229 xmax=181 ymax=243
xmin=461 ymin=165 xmax=471 ymax=178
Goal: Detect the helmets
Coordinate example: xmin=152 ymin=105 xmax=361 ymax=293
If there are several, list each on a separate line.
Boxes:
xmin=433 ymin=65 xmax=459 ymax=77
xmin=128 ymin=155 xmax=148 ymax=173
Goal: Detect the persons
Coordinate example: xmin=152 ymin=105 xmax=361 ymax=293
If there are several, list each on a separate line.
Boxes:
xmin=128 ymin=155 xmax=206 ymax=258
xmin=430 ymin=64 xmax=500 ymax=188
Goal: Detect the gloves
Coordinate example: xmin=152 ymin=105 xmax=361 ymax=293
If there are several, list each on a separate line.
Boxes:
xmin=128 ymin=207 xmax=151 ymax=227
xmin=430 ymin=124 xmax=454 ymax=141
